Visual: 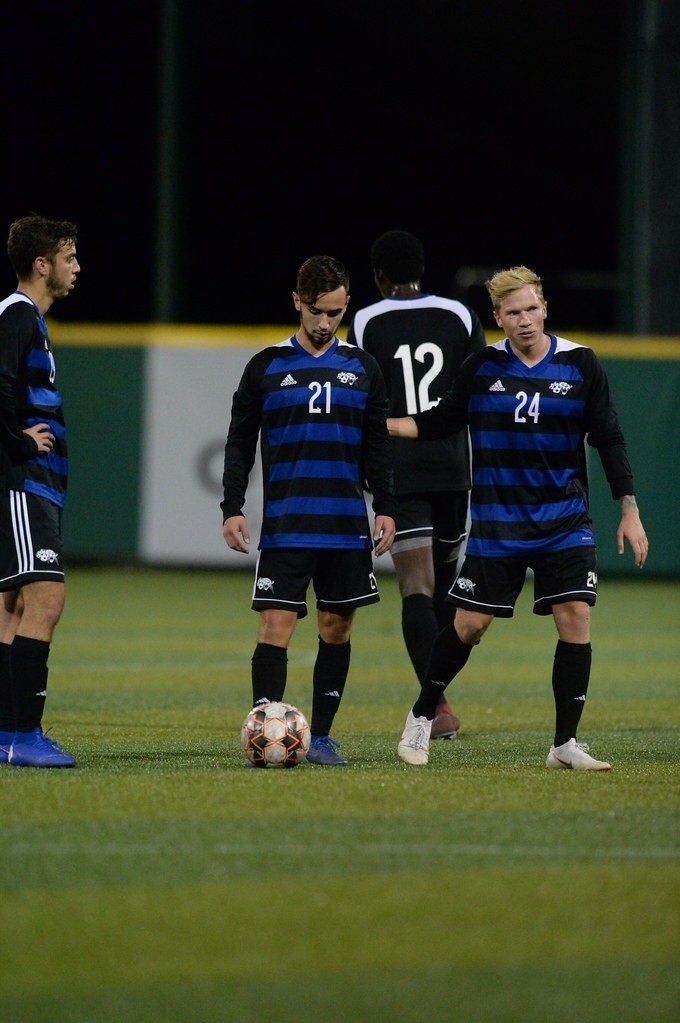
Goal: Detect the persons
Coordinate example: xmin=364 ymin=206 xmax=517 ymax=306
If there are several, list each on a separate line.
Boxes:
xmin=1 ymin=217 xmax=81 ymax=768
xmin=386 ymin=264 xmax=650 ymax=771
xmin=346 ymin=234 xmax=486 ymax=738
xmin=219 ymin=256 xmax=397 ymax=765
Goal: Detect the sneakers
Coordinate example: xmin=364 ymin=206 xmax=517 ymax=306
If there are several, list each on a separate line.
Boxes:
xmin=546 ymin=737 xmax=612 ymax=769
xmin=306 ymin=737 xmax=348 ymax=764
xmin=431 ymin=712 xmax=459 ymax=739
xmin=0 ymin=729 xmax=76 ymax=767
xmin=398 ymin=708 xmax=433 ymax=765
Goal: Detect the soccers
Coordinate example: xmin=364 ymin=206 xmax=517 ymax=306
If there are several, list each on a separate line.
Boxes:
xmin=240 ymin=702 xmax=311 ymax=769
